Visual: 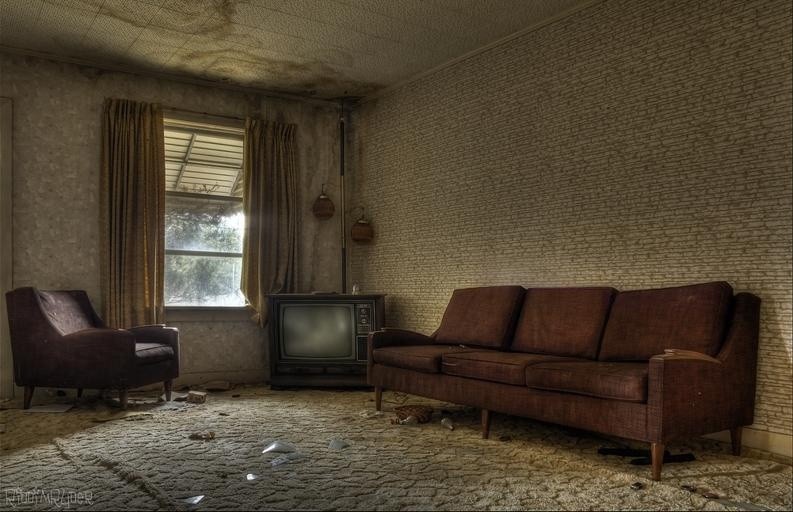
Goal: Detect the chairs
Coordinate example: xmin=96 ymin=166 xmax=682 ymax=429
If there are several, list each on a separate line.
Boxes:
xmin=5 ymin=286 xmax=178 ymax=411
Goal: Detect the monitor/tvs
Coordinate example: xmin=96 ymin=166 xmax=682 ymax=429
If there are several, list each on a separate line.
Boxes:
xmin=278 ymin=299 xmax=376 ymax=371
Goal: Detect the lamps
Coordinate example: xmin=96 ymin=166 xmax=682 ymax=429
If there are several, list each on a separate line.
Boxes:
xmin=311 ymin=100 xmax=373 ymax=293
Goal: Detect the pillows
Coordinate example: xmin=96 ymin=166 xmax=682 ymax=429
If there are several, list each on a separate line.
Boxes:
xmin=598 ymin=281 xmax=733 ymax=363
xmin=434 ymin=286 xmax=526 ymax=349
xmin=510 ymin=287 xmax=619 ymax=361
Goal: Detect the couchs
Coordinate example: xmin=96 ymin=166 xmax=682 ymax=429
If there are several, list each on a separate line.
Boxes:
xmin=366 ymin=292 xmax=761 ymax=482
